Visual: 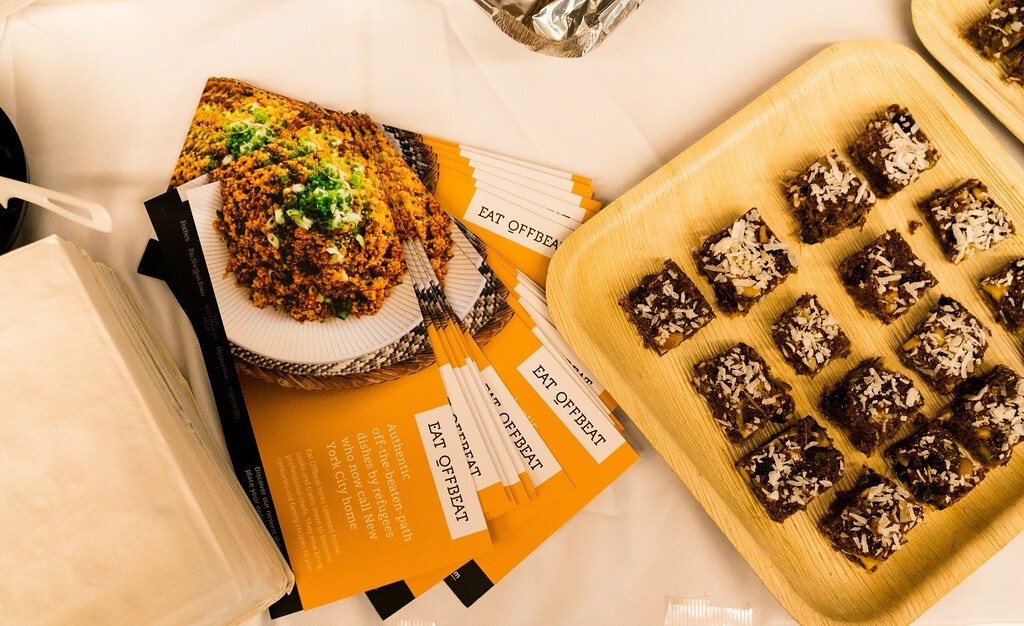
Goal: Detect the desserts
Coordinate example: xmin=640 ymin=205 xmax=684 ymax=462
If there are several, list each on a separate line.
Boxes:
xmin=618 ymin=0 xmax=1023 ymax=572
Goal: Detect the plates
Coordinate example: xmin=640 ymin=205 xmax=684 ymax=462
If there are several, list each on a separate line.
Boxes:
xmin=187 ymin=179 xmax=432 ymax=377
xmin=908 ymin=0 xmax=1023 ymax=146
xmin=2 ymin=107 xmax=36 ymax=255
xmin=550 ymin=33 xmax=1023 ymax=623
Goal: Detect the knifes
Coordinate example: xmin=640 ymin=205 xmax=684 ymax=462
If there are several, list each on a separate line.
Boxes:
xmin=0 ymin=171 xmax=114 ymax=236
